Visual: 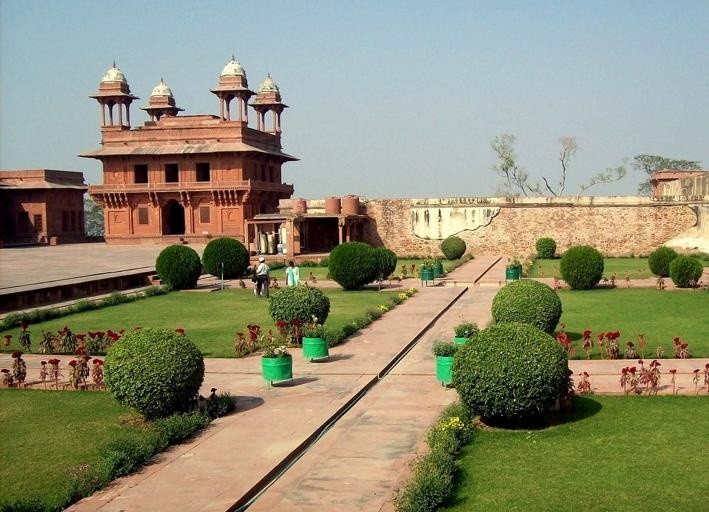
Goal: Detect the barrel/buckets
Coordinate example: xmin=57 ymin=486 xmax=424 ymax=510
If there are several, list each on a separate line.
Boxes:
xmin=435 ymin=355 xmax=455 ymax=386
xmin=454 ymin=336 xmax=469 ymax=345
xmin=262 ymin=353 xmax=293 ymax=384
xmin=302 ymin=337 xmax=328 ymax=360
xmin=431 ymin=265 xmax=443 ymax=276
xmin=506 ymin=263 xmax=522 ymax=280
xmin=421 ymin=268 xmax=433 ymax=280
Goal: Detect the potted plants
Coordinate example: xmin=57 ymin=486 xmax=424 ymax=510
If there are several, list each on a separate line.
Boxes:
xmin=506 ymin=256 xmax=522 ymax=279
xmin=259 ymin=344 xmax=294 ymax=389
xmin=432 ymin=321 xmax=481 ymax=389
xmin=421 ymin=255 xmax=443 ymax=280
xmin=302 ymin=325 xmax=329 ymax=363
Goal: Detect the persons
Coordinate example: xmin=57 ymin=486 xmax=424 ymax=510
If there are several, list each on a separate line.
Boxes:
xmin=285 ymin=261 xmax=300 ymax=288
xmin=253 ymin=256 xmax=270 ymax=298
